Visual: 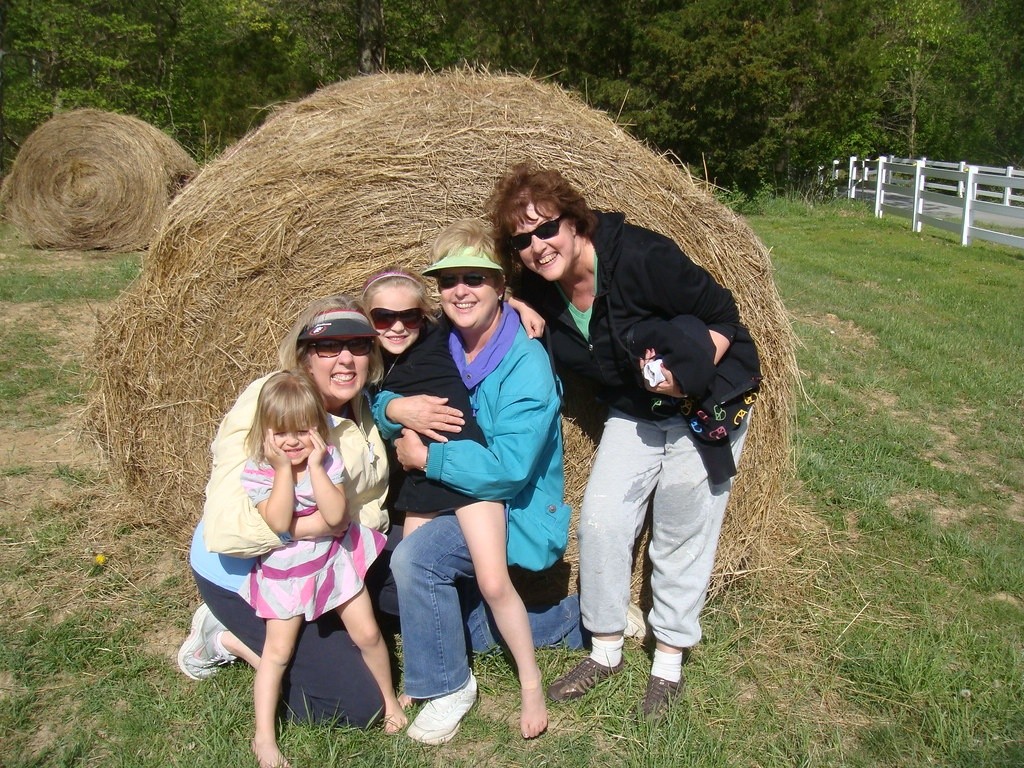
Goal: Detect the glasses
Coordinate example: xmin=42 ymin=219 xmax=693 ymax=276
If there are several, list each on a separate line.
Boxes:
xmin=437 ymin=271 xmax=487 ymax=288
xmin=308 ymin=337 xmax=372 ymax=358
xmin=508 ymin=212 xmax=567 ymax=250
xmin=370 ymin=308 xmax=424 ymax=329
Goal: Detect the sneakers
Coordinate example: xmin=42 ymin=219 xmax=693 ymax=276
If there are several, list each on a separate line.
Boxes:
xmin=624 ymin=602 xmax=654 ymax=639
xmin=407 ymin=667 xmax=478 ymax=745
xmin=546 ymin=653 xmax=624 ymax=703
xmin=643 ymin=667 xmax=683 ymax=726
xmin=178 ymin=602 xmax=237 ymax=681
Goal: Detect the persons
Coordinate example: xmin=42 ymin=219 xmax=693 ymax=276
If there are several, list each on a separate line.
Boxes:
xmin=494 ymin=164 xmax=764 ymax=729
xmin=179 ymin=220 xmax=653 ymax=768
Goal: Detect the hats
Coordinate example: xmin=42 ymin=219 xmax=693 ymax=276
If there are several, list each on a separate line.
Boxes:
xmin=421 ymin=245 xmax=503 ymax=276
xmin=298 ymin=310 xmax=381 ymax=340
